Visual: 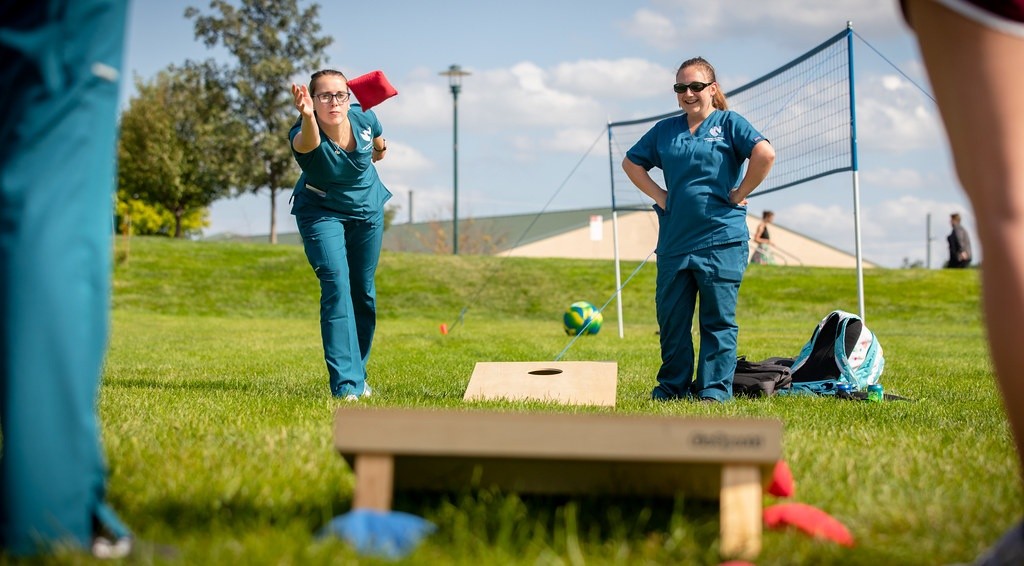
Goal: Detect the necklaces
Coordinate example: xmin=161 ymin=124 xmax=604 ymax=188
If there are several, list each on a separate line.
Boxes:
xmin=327 ymin=125 xmax=345 ymax=154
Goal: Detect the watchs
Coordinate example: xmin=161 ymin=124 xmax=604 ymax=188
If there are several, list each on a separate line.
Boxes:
xmin=374 ymin=139 xmax=386 ymax=151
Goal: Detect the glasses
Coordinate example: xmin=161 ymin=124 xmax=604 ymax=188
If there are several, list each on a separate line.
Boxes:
xmin=313 ymin=92 xmax=351 ymax=103
xmin=674 ymin=82 xmax=714 ymax=93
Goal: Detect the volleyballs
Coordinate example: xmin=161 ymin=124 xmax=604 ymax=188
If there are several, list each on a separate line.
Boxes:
xmin=563 ymin=301 xmax=604 ymax=336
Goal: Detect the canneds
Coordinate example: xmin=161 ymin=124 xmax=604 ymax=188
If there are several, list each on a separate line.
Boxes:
xmin=835 ymin=382 xmax=851 ymax=400
xmin=867 ymin=384 xmax=884 ymax=403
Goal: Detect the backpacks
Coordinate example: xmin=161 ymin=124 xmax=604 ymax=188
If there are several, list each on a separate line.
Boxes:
xmin=691 ymin=356 xmax=793 ymax=399
xmin=777 ymin=311 xmax=885 ymax=397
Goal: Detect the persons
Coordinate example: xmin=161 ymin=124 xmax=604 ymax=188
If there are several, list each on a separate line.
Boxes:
xmin=898 ymin=0 xmax=1024 ymax=566
xmin=947 ymin=214 xmax=971 ymax=268
xmin=289 ymin=69 xmax=393 ymax=402
xmin=751 ymin=211 xmax=776 ymax=265
xmin=623 ymin=58 xmax=775 ymax=404
xmin=0 ymin=0 xmax=131 ymax=556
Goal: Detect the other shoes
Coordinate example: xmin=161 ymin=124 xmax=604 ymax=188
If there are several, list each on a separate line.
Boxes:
xmin=363 ymin=381 xmax=373 ymax=396
xmin=343 ymin=395 xmax=358 ymax=402
